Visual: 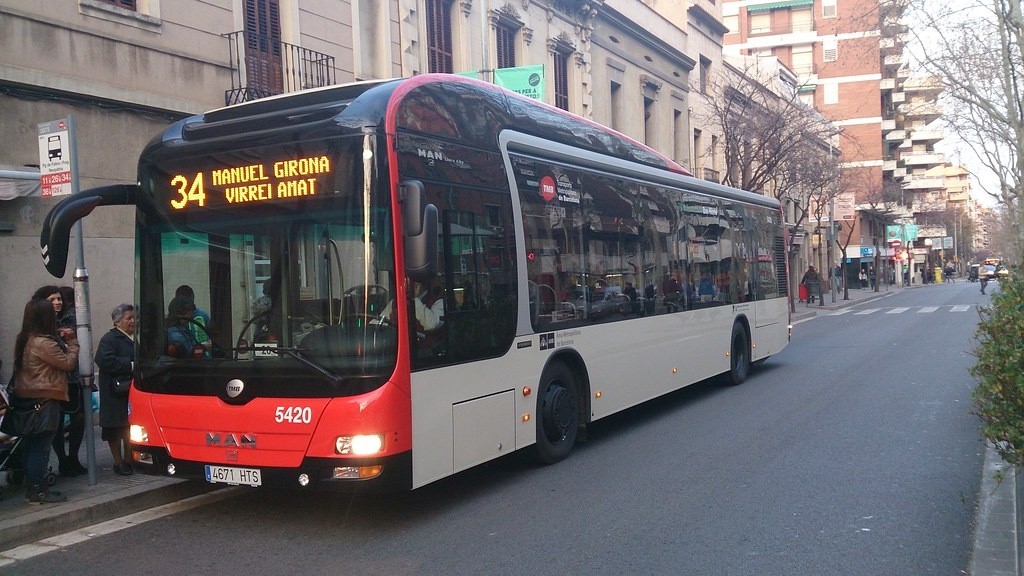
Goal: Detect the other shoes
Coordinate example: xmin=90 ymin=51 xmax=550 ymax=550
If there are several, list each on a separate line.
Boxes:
xmin=113 ymin=463 xmax=133 ymax=475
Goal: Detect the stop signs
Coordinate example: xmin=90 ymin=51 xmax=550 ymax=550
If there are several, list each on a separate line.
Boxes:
xmin=892 ymin=240 xmax=901 ymax=249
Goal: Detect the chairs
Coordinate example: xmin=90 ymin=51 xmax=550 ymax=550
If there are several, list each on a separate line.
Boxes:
xmin=518 ymin=271 xmax=739 ymax=331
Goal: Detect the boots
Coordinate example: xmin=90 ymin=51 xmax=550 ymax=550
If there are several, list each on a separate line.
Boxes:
xmin=65 ymin=455 xmax=88 ymax=477
xmin=29 ymin=479 xmax=66 ymax=505
xmin=23 ymin=479 xmax=60 ymax=503
xmin=57 ymin=455 xmax=68 ymax=474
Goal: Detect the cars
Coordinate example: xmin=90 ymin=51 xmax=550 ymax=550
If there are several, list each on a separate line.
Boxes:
xmin=968 ymin=257 xmax=1009 ymax=281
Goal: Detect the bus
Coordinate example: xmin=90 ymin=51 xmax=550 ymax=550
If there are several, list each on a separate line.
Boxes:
xmin=38 ymin=72 xmax=793 ymax=503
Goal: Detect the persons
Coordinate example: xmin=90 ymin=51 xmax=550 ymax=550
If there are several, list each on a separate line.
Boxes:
xmin=698 ymin=272 xmax=715 ymax=302
xmin=623 ymin=282 xmax=637 ymax=316
xmin=801 ymin=265 xmax=818 ymax=303
xmin=947 ymin=266 xmax=955 ymax=283
xmin=369 ymin=281 xmax=444 ymax=349
xmin=829 ymin=260 xmax=843 ymax=294
xmin=167 ymin=296 xmax=212 ymax=357
xmin=978 ymin=261 xmax=989 ymax=294
xmin=870 ymin=265 xmax=875 ymax=290
xmin=32 ymin=286 xmax=88 ymax=476
xmin=175 ymin=285 xmax=212 ymax=357
xmin=662 ymin=272 xmax=679 ymax=313
xmin=541 ymin=236 xmax=567 ymax=302
xmin=94 ymin=303 xmax=135 ymax=474
xmin=859 ymin=268 xmax=868 ymax=290
xmin=14 ymin=297 xmax=80 ymax=505
xmin=251 ymin=277 xmax=273 ymax=340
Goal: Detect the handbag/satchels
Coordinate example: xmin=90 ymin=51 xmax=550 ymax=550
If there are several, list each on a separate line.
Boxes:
xmin=0 ymin=405 xmax=39 ymax=438
xmin=111 ymin=374 xmax=134 ymax=396
xmin=62 ymin=403 xmax=85 ymax=430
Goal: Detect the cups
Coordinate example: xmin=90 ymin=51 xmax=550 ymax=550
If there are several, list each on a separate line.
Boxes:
xmin=453 ymin=288 xmax=464 ymax=308
